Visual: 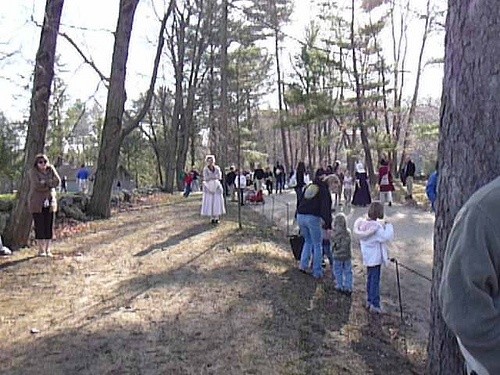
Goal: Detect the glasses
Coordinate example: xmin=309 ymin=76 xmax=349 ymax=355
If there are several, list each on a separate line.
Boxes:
xmin=39 ymin=160 xmax=47 ymax=164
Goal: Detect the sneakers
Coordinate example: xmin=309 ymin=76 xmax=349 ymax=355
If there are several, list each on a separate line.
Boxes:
xmin=366 ymin=301 xmax=386 ymax=314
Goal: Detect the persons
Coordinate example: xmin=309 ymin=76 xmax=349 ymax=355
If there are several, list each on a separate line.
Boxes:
xmin=183 ymin=155 xmax=437 ymax=314
xmin=437 ymin=177 xmax=500 ymax=375
xmin=26 ymin=153 xmax=122 ymax=257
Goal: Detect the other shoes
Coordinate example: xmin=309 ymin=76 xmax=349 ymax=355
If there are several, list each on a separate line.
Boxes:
xmin=299 ymin=265 xmax=310 ymax=273
xmin=312 ymin=271 xmax=323 ymax=279
xmin=210 ymin=217 xmax=219 ymax=223
xmin=341 ymin=286 xmax=352 ymax=295
xmin=334 ymin=284 xmax=344 ymax=291
xmin=39 ymin=252 xmax=53 ymax=257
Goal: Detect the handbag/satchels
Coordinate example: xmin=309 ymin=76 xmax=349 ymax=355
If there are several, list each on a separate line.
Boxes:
xmin=290 ymin=234 xmax=305 ymax=260
